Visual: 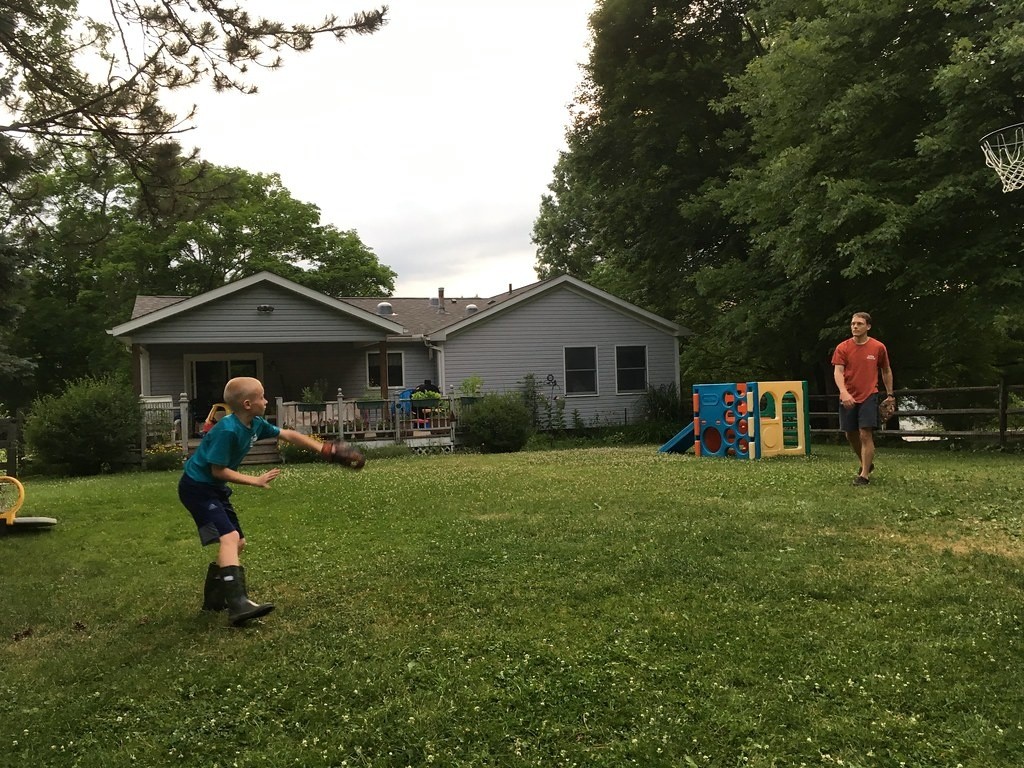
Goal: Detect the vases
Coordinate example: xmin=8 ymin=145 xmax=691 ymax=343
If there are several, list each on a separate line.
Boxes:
xmin=281 ymin=452 xmax=315 ymax=464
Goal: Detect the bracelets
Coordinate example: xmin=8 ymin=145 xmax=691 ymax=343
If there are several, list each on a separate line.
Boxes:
xmin=887 ymin=393 xmax=895 ymax=398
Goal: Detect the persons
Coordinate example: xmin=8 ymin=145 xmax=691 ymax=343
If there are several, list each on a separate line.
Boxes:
xmin=832 ymin=311 xmax=895 ymax=485
xmin=177 ymin=377 xmax=365 ymax=625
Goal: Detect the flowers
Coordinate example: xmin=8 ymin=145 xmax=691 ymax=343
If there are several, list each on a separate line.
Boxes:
xmin=275 ymin=432 xmax=324 ymax=454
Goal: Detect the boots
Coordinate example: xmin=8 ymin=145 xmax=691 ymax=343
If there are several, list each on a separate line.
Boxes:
xmin=201 ymin=562 xmax=229 ymax=614
xmin=220 ymin=565 xmax=275 ymax=625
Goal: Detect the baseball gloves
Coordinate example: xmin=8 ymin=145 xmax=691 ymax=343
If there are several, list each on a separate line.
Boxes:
xmin=320 ymin=438 xmax=368 ymax=471
xmin=878 ymin=395 xmax=896 ymax=424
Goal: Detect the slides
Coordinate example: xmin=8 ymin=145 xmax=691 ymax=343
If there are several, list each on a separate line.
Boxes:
xmin=655 ymin=419 xmax=699 ymax=455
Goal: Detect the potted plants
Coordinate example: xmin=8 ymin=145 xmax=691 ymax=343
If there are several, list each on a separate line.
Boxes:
xmin=341 ymin=417 xmax=390 ymax=439
xmin=459 ymin=372 xmax=484 ymax=406
xmin=298 ymin=379 xmax=452 ymax=442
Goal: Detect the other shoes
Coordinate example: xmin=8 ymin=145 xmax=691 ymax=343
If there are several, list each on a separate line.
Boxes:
xmin=859 ymin=463 xmax=874 ymax=475
xmin=853 ymin=476 xmax=869 ymax=486
